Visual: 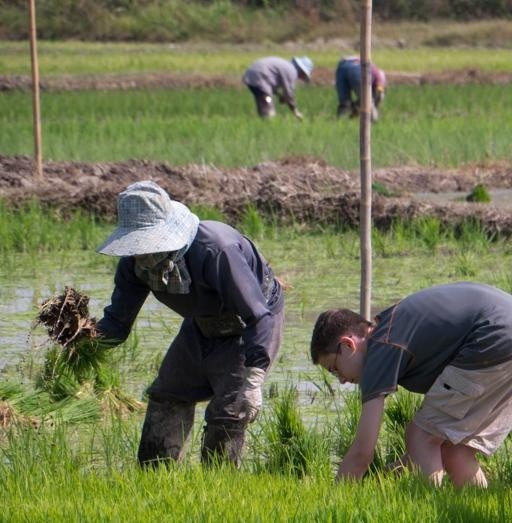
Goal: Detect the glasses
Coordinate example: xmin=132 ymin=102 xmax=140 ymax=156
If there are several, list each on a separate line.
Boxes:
xmin=330 ymin=335 xmax=352 ymax=378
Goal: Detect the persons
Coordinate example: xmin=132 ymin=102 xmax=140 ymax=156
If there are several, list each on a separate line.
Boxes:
xmin=46 ymin=178 xmax=285 ymax=468
xmin=242 ymin=54 xmax=313 ymax=121
xmin=306 ymin=280 xmax=512 ymax=490
xmin=335 ymin=55 xmax=387 ymax=123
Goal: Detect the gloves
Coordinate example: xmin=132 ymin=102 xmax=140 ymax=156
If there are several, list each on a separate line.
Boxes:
xmin=234 ymin=367 xmax=267 ymax=423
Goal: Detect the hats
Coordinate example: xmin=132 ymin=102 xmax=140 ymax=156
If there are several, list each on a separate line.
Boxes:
xmin=94 ymin=180 xmax=199 ymax=257
xmin=292 ymin=55 xmax=315 ymax=82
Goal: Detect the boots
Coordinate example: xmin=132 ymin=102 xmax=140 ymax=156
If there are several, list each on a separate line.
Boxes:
xmin=138 ymin=396 xmax=195 ymax=472
xmin=198 ymin=419 xmax=245 ymax=470
